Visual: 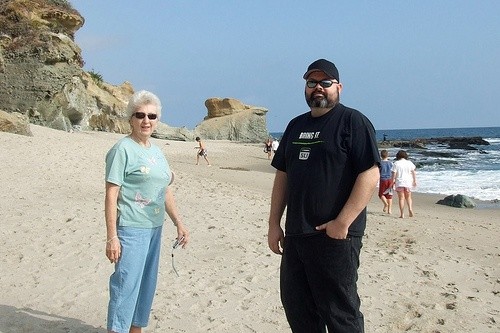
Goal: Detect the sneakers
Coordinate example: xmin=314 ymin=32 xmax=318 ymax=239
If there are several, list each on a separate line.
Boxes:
xmin=383 ymin=188 xmax=393 ymax=195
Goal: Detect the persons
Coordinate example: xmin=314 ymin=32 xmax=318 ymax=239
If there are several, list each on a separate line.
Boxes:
xmin=263 ymin=137 xmax=279 ymax=159
xmin=194 ymin=136 xmax=210 ymax=165
xmin=378 ymin=149 xmax=394 ymax=214
xmin=382 ymin=132 xmax=388 ymax=141
xmin=390 ymin=149 xmax=416 ymax=217
xmin=105 ymin=90 xmax=189 ymax=332
xmin=268 ymin=57 xmax=379 ymax=333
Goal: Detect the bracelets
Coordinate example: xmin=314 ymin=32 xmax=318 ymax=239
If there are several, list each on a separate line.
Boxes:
xmin=106 ymin=235 xmax=118 ymax=244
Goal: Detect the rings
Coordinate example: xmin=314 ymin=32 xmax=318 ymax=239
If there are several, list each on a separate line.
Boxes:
xmin=110 ymin=252 xmax=115 ymax=255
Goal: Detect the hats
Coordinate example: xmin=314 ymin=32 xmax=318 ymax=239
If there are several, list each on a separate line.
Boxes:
xmin=303 ymin=59 xmax=339 ymax=84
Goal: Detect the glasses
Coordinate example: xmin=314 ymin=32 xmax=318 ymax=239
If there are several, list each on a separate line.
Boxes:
xmin=306 ymin=79 xmax=338 ymax=88
xmin=129 ymin=112 xmax=158 ymax=120
